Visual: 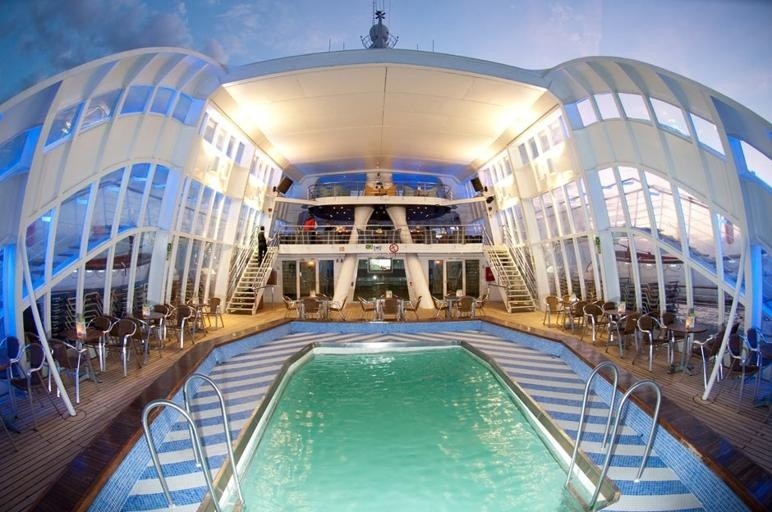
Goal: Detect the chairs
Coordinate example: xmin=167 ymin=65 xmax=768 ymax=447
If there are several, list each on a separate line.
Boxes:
xmin=279 ymin=288 xmax=490 ymax=321
xmin=293 ymin=225 xmax=464 ymax=244
xmin=0 ymin=297 xmax=224 ymax=450
xmin=544 ymin=293 xmax=772 ymax=389
xmin=320 ymin=185 xmax=438 ymax=196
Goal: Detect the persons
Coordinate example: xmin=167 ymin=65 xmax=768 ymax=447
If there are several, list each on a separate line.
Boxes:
xmin=257 ymin=226 xmax=269 ymax=267
xmin=304 ymin=216 xmax=319 ymax=243
